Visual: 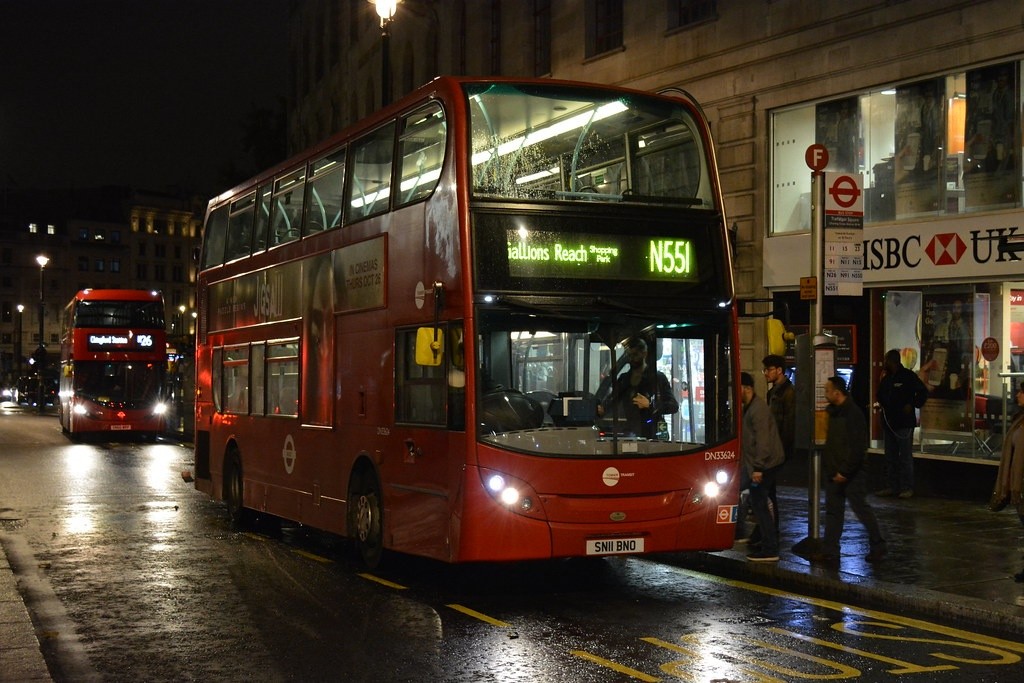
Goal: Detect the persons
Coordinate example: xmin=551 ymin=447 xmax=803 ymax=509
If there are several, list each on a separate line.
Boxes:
xmin=876 ymin=350 xmax=928 ymax=500
xmin=991 ymin=382 xmax=1024 ymax=582
xmin=429 ymin=327 xmax=506 ymax=428
xmin=598 ymin=338 xmax=680 ymax=441
xmin=741 ymin=371 xmax=786 ymax=560
xmin=808 ymin=376 xmax=888 ymax=562
xmin=747 ymin=355 xmax=796 ymax=546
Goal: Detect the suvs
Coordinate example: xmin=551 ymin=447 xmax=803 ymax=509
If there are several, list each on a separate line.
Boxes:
xmin=16 ymin=376 xmax=56 ymax=407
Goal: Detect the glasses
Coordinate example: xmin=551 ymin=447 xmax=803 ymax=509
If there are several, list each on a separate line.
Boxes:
xmin=761 ymin=368 xmax=777 ymax=374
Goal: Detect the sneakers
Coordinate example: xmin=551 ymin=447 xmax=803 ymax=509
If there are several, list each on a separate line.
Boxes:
xmin=865 ymin=549 xmax=888 ymax=562
xmin=747 ymin=542 xmax=780 ymax=562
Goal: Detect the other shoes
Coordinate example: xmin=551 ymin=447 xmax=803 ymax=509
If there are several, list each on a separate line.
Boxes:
xmin=735 ymin=528 xmax=749 ymax=543
xmin=874 ymin=487 xmax=916 ymax=499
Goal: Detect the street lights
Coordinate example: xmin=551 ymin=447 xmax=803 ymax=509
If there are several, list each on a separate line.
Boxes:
xmin=36 ymin=255 xmax=50 ymax=411
xmin=368 ymin=0 xmax=401 ymax=107
xmin=17 ymin=304 xmax=23 ymax=376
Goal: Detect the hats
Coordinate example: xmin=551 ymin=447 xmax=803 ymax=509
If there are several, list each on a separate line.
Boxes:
xmin=728 ymin=372 xmax=756 ymax=387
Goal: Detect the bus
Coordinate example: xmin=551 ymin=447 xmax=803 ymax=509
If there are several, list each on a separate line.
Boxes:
xmin=191 ymin=67 xmax=792 ymax=567
xmin=59 ymin=289 xmax=178 ymax=437
